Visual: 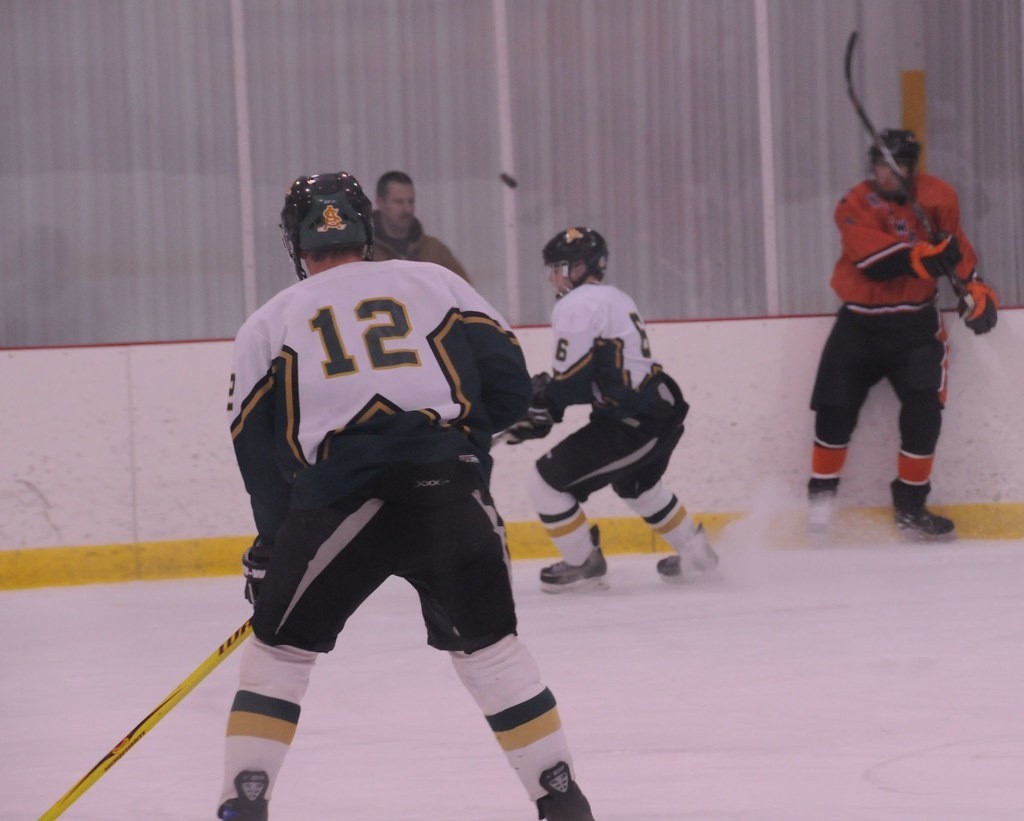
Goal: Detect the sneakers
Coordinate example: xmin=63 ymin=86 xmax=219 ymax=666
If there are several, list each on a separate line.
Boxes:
xmin=537 ymin=761 xmax=595 ymax=821
xmin=539 ymin=524 xmax=607 ymax=594
xmin=218 ymin=770 xmax=268 ymax=821
xmin=807 ymin=480 xmax=839 ymax=538
xmin=656 ymin=524 xmax=718 ymax=582
xmin=891 ymin=479 xmax=955 ymax=542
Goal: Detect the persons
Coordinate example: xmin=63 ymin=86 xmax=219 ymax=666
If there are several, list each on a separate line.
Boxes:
xmin=215 ymin=172 xmax=592 ymax=821
xmin=507 ymin=227 xmax=725 ymax=596
xmin=364 ymin=170 xmax=467 ymax=285
xmin=806 ymin=129 xmax=999 ymax=537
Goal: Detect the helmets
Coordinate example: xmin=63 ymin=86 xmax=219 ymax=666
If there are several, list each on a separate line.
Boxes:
xmin=543 ymin=226 xmax=608 ymax=280
xmin=866 ymin=128 xmax=921 ymax=200
xmin=278 ymin=172 xmax=374 ymax=258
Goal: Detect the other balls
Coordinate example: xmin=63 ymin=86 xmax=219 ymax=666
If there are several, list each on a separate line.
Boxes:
xmin=500 ymin=172 xmax=518 ymax=189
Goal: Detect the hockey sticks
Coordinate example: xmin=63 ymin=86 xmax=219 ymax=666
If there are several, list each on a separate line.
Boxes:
xmin=843 ymin=29 xmax=976 ymax=310
xmin=36 ymin=616 xmax=256 ymax=821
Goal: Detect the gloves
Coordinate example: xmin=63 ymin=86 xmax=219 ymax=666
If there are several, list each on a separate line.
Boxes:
xmin=529 ymin=373 xmax=553 ymax=409
xmin=912 ymin=234 xmax=964 ymax=280
xmin=243 ymin=539 xmax=273 ymax=604
xmin=507 ymin=408 xmax=553 ymax=445
xmin=958 ymin=281 xmax=997 ymax=335
